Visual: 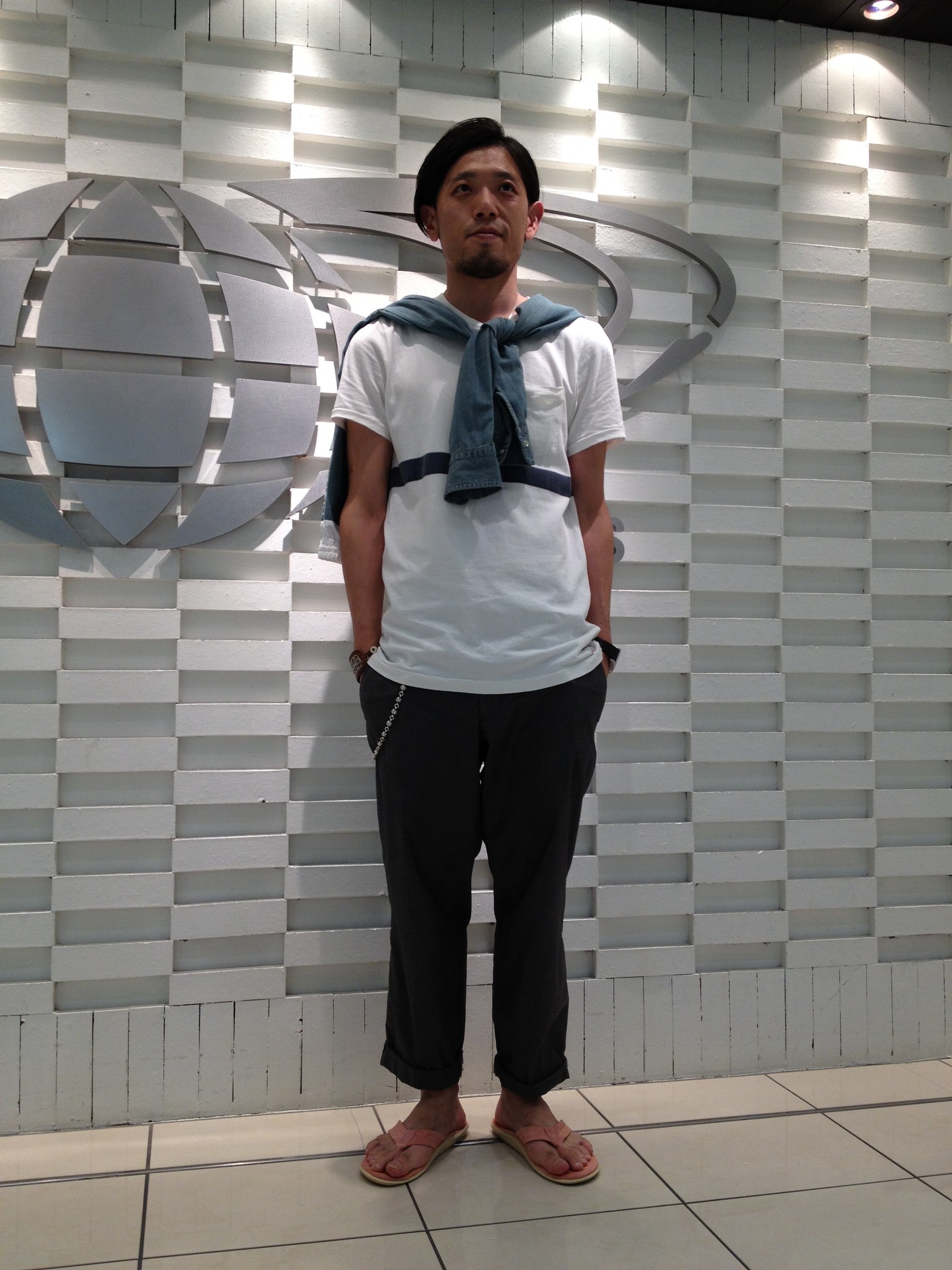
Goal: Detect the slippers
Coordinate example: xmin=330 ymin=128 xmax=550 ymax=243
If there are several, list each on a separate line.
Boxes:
xmin=491 ymin=1118 xmax=599 ymax=1184
xmin=360 ymin=1119 xmax=470 ymax=1186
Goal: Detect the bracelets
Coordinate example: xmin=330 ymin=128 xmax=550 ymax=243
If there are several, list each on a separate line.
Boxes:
xmin=349 ymin=645 xmax=379 ymax=681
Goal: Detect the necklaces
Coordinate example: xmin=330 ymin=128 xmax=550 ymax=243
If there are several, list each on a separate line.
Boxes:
xmin=509 ymin=296 xmax=518 ymax=320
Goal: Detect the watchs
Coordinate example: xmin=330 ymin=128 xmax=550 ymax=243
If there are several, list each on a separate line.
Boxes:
xmin=593 ymin=636 xmax=621 ymax=674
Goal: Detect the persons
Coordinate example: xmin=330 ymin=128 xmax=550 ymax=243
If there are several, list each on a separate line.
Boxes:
xmin=328 ymin=119 xmax=628 ymax=1186
